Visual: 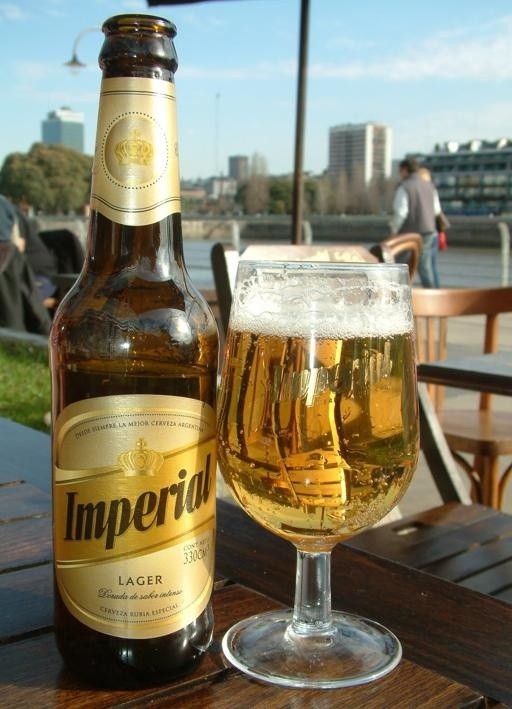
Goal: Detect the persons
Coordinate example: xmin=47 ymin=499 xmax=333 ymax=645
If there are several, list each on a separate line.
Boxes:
xmin=0 ymin=192 xmax=52 ymax=338
xmin=389 ymin=158 xmax=450 ymax=290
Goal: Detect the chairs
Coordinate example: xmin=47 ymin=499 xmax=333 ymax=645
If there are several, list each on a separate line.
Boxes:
xmin=411 ymin=285 xmax=510 ymax=511
xmin=370 ymin=232 xmax=424 ymax=286
xmin=211 ymin=240 xmax=238 ymax=328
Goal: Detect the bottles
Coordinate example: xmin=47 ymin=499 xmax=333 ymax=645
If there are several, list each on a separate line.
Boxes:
xmin=46 ymin=11 xmax=222 ymax=690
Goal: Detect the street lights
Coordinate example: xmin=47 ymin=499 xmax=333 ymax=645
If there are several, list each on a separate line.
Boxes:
xmin=61 ymin=27 xmax=100 ymax=77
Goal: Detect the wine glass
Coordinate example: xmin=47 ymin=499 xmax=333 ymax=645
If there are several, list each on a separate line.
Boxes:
xmin=215 ymin=260 xmax=423 ymax=691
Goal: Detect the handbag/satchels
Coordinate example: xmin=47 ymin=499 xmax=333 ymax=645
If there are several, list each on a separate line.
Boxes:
xmin=436 ymin=213 xmax=450 ymax=232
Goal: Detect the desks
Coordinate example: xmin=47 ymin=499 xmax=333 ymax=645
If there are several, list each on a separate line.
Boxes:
xmin=416 ymin=350 xmax=511 ymax=400
